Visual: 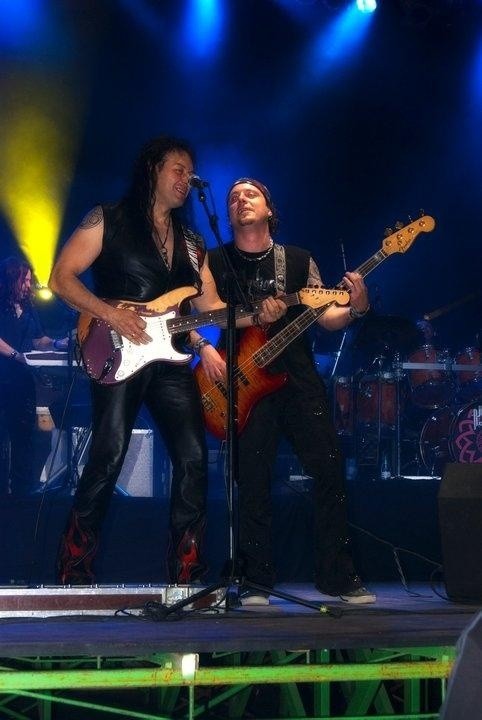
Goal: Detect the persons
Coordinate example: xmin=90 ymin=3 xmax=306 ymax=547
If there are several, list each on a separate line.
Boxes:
xmin=45 ymin=132 xmax=286 ymax=589
xmin=189 ymin=174 xmax=382 ymax=607
xmin=0 ymin=257 xmax=69 ymax=498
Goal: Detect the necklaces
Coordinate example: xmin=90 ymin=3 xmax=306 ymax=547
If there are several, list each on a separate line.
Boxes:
xmin=150 ymin=215 xmax=172 ymax=269
xmin=232 ymin=239 xmax=275 ymax=263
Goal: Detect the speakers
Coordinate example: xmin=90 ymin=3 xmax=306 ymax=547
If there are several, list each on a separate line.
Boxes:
xmin=437 ymin=462 xmax=481 ymax=607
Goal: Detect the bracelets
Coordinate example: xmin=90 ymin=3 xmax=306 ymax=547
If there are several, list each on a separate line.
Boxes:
xmin=351 ymin=307 xmax=374 ymax=320
xmin=7 ymin=351 xmax=18 ymax=360
xmin=254 ymin=315 xmax=269 ymax=336
xmin=193 ymin=337 xmax=211 ymax=353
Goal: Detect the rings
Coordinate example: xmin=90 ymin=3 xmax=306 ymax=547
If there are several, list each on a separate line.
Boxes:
xmin=130 ymin=331 xmax=139 ymax=337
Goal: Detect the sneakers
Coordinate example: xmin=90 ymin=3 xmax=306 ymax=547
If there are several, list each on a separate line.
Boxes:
xmin=339 ymin=587 xmax=377 ymax=603
xmin=240 ymin=589 xmax=269 ymax=606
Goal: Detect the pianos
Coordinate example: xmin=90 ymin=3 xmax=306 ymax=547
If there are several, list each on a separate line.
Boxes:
xmin=24 ymin=349 xmax=90 ymax=388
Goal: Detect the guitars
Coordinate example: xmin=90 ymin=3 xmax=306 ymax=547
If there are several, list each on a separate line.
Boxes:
xmin=76 ymin=285 xmax=352 ymax=387
xmin=194 ymin=208 xmax=437 ymax=439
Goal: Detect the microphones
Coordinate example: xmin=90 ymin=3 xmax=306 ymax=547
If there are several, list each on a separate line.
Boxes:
xmin=35 ymin=283 xmax=50 ymax=292
xmin=186 ymin=174 xmax=211 ymax=189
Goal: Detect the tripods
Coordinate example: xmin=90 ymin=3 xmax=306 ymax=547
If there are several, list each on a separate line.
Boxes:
xmin=151 ymin=188 xmax=345 ymax=621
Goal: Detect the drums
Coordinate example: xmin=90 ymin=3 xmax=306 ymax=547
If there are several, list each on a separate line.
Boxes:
xmin=419 ymin=398 xmax=481 ymax=478
xmin=406 ymin=344 xmax=458 ymax=410
xmin=330 ymin=377 xmax=353 ymax=435
xmin=358 ymin=372 xmax=406 ymax=425
xmin=451 ymin=347 xmax=481 ymax=395
